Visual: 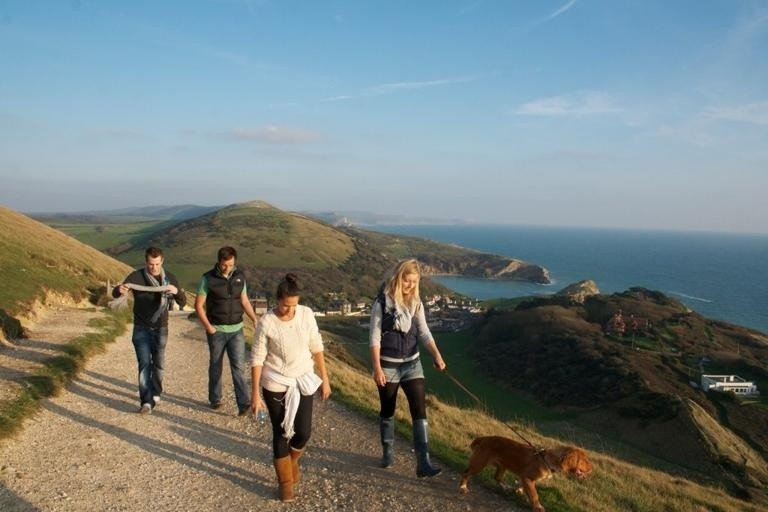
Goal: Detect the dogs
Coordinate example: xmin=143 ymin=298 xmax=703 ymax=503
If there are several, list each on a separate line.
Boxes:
xmin=459 ymin=436 xmax=593 ymax=512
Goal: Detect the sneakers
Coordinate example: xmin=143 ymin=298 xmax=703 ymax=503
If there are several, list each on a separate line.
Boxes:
xmin=240 ymin=405 xmax=253 ymax=416
xmin=141 ymin=401 xmax=152 ymax=415
xmin=211 ymin=400 xmax=225 ymax=410
xmin=153 ymin=396 xmax=161 ymax=404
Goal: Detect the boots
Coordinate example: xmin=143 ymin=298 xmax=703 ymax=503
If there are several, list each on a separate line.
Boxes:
xmin=412 ymin=419 xmax=442 ymax=477
xmin=289 ymin=445 xmax=305 ymax=485
xmin=378 ymin=415 xmax=394 ymax=467
xmin=273 ymin=453 xmax=295 ymax=503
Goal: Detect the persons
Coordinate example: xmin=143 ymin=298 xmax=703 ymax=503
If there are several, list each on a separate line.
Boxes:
xmin=113 ymin=247 xmax=188 ymax=419
xmin=194 ymin=245 xmax=259 ymax=418
xmin=248 ymin=272 xmax=333 ymax=504
xmin=368 ymin=256 xmax=449 ymax=479
